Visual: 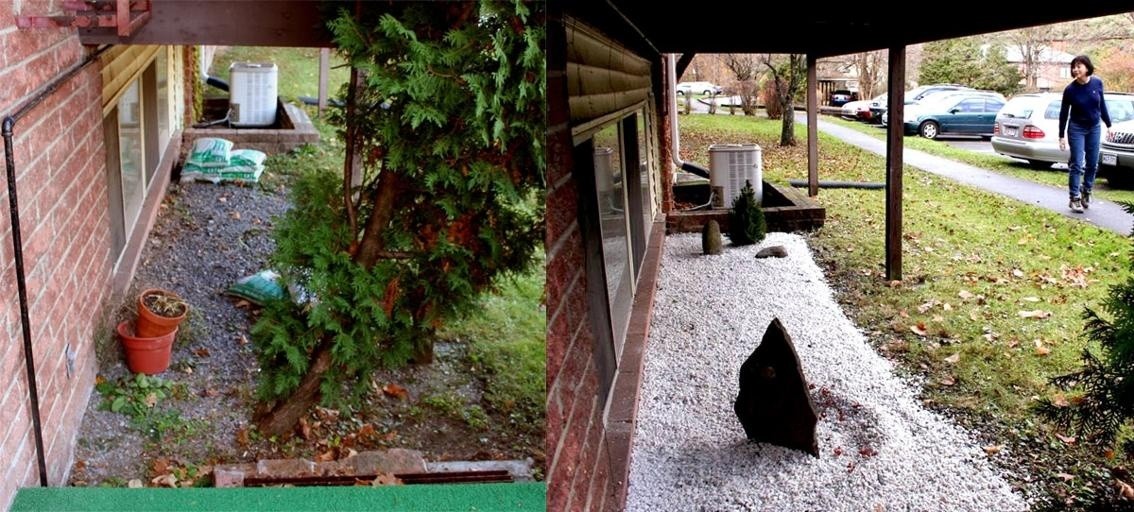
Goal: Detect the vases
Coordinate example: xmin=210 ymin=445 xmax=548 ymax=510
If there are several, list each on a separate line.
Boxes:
xmin=138 ymin=287 xmax=188 ymax=338
xmin=118 ymin=320 xmax=179 ymax=372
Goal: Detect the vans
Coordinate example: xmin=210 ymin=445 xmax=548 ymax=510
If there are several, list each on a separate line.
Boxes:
xmin=990 ymin=91 xmax=1133 ymax=178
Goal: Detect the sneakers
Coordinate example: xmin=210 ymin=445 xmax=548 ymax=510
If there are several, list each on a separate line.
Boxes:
xmin=1080 ymin=193 xmax=1090 ymax=208
xmin=1069 ymin=201 xmax=1083 ymax=212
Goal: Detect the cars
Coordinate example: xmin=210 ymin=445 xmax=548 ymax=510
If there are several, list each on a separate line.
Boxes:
xmin=675 ymin=82 xmax=717 ymax=96
xmin=840 ymin=88 xmax=889 ymax=122
xmin=706 ymin=82 xmax=721 ymax=95
xmin=881 ymin=90 xmax=1007 ymax=141
xmin=830 ymin=90 xmax=856 ymax=106
xmin=1098 ymin=119 xmax=1133 ymax=189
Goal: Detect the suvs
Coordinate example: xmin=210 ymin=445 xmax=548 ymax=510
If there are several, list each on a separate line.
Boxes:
xmin=882 ymin=82 xmax=974 ymax=111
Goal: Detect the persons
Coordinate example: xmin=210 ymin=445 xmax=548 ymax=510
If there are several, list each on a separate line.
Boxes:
xmin=1056 ymin=54 xmax=1114 ymax=214
